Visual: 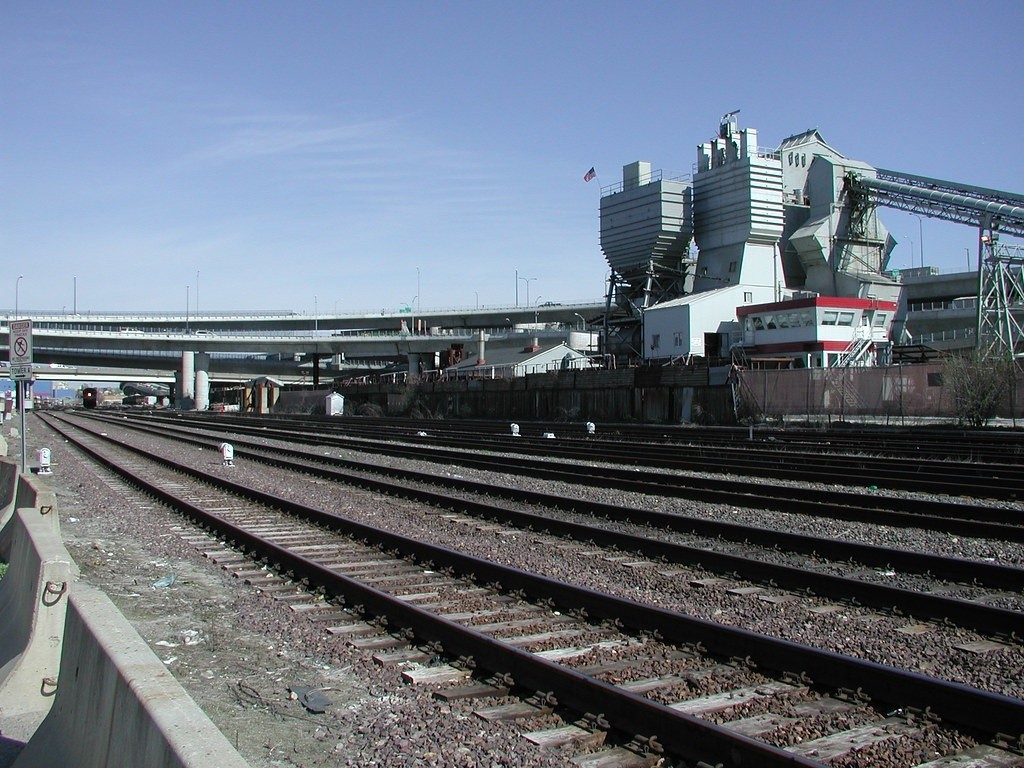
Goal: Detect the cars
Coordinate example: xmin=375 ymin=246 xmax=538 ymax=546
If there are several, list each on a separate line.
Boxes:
xmin=196 ymin=331 xmax=215 ymax=336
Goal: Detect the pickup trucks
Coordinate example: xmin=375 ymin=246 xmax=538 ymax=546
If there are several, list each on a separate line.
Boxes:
xmin=538 ymin=301 xmax=561 ymax=307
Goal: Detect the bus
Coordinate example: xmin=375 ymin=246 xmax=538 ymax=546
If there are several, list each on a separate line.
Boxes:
xmin=121 ymin=394 xmax=145 ymax=408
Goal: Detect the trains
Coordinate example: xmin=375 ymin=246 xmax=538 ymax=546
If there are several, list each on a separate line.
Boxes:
xmin=83 ymin=388 xmax=97 ymax=409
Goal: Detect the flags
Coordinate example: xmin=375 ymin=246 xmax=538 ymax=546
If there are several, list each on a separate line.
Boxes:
xmin=584 ymin=167 xmax=596 ymax=182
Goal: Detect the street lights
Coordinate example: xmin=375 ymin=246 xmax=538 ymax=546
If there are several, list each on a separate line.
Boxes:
xmin=314 ymin=295 xmax=318 ymax=337
xmin=15 ymin=276 xmax=23 ymax=319
xmin=909 ymin=213 xmax=925 ymax=267
xmin=575 ymin=313 xmax=586 ymax=331
xmin=519 ymin=278 xmax=537 ymax=307
xmin=415 ymin=266 xmax=421 ymax=335
xmin=515 ymin=269 xmax=518 ymax=306
xmin=186 ymin=285 xmax=189 ymax=334
xmin=534 ymin=296 xmax=541 ymax=332
xmin=197 ymin=270 xmax=200 ymax=316
xmin=904 ymin=236 xmax=913 ymax=268
xmin=411 ymin=296 xmax=418 ymax=336
xmin=965 ymin=248 xmax=970 ymax=272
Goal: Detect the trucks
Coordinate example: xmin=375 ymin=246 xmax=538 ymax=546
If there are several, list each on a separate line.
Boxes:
xmin=430 ymin=327 xmax=447 ymax=336
